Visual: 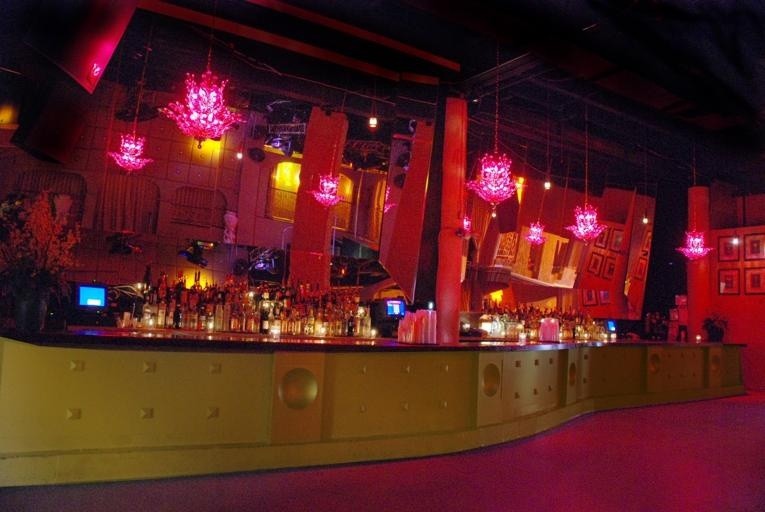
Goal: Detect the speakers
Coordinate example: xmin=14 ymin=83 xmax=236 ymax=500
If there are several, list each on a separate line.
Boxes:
xmin=8 ymin=72 xmax=90 ymax=164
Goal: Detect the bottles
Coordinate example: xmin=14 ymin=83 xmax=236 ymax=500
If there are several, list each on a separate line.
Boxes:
xmin=116 ymin=268 xmax=374 ymax=337
xmin=475 ymin=300 xmax=610 ymax=344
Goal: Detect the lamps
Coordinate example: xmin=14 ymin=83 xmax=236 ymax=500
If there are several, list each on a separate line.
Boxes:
xmin=308 ymin=86 xmax=347 ymax=210
xmin=673 ymin=140 xmax=716 ymax=261
xmin=565 ymin=101 xmax=607 ymax=244
xmin=526 ymin=163 xmax=547 ymax=245
xmin=106 ymin=2 xmax=250 ymax=179
xmin=464 ymin=45 xmax=519 ymax=208
xmin=463 ymin=210 xmax=471 ymax=234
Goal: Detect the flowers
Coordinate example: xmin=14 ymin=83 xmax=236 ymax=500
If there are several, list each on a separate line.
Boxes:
xmin=0 ymin=186 xmax=88 ymax=281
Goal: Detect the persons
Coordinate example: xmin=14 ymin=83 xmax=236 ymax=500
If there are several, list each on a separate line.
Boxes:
xmin=647 ymin=318 xmax=669 ymax=341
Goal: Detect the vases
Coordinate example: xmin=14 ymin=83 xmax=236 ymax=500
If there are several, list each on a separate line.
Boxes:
xmin=0 ymin=255 xmax=74 ymax=343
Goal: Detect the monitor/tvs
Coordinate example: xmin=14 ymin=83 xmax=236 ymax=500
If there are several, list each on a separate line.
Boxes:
xmin=607 ymin=319 xmax=616 ymax=332
xmin=385 ymin=298 xmax=406 ymax=318
xmin=75 ymin=282 xmax=108 ymax=311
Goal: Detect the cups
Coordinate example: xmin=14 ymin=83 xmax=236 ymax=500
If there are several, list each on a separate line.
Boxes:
xmin=397 ymin=309 xmax=437 ymax=345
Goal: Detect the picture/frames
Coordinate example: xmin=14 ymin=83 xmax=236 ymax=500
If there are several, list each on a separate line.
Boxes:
xmin=717 ymin=232 xmax=765 ymax=296
